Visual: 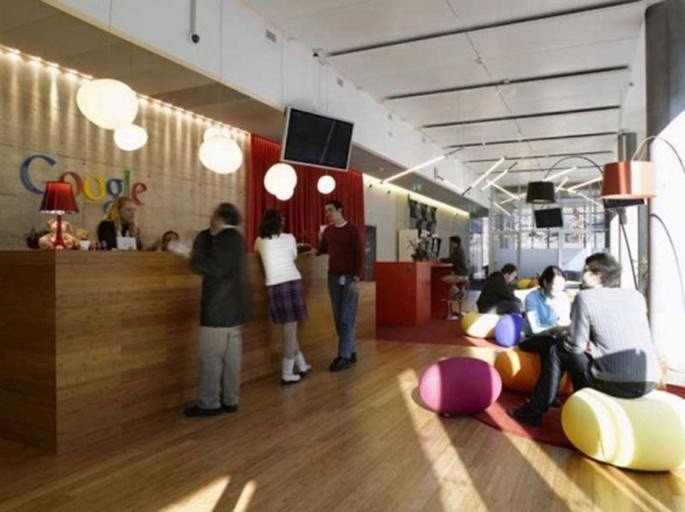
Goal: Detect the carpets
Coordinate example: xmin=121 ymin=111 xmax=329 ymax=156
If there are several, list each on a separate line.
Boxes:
xmin=372 ymin=309 xmax=499 ymax=349
xmin=470 ymin=380 xmax=575 ymax=446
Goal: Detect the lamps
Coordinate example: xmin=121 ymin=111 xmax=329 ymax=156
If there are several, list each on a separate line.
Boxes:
xmin=74 ymin=1 xmax=138 ymax=132
xmin=316 ymin=61 xmax=337 ymax=197
xmin=111 ymin=43 xmax=150 ymax=155
xmin=599 ymin=197 xmax=685 ymax=328
xmin=192 ymin=3 xmax=246 ymax=179
xmin=525 ymin=153 xmax=610 ymax=206
xmin=263 ymin=30 xmax=297 ymax=198
xmin=37 ymin=178 xmax=80 ymax=249
xmin=598 ymin=133 xmax=685 ymax=210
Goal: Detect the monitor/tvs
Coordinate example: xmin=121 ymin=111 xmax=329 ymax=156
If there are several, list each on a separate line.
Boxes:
xmin=535 ymin=208 xmax=563 ymax=227
xmin=279 ymin=106 xmax=355 ymax=173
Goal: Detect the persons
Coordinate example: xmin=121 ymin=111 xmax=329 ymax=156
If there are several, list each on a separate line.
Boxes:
xmin=504 ymin=253 xmax=659 ymax=426
xmin=183 ymin=202 xmax=259 ymax=417
xmin=302 ymin=200 xmax=366 ymax=371
xmin=520 ymin=266 xmax=594 ymax=405
xmin=96 ymin=198 xmax=162 ymax=250
xmin=435 ymin=236 xmax=469 ymax=300
xmin=475 ymin=265 xmax=524 ymax=316
xmin=252 ymin=208 xmax=312 ymax=384
xmin=155 ymin=231 xmax=180 ymax=249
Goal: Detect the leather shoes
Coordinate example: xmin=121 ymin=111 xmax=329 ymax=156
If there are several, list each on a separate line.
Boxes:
xmin=330 ymin=356 xmax=350 ymax=372
xmin=333 ymin=352 xmax=357 ymax=363
xmin=507 ymin=400 xmax=541 ymax=427
xmin=221 ymin=401 xmax=238 ymax=413
xmin=183 ymin=404 xmax=222 ymax=416
xmin=281 ymin=374 xmax=301 ymax=385
xmin=299 ymin=364 xmax=313 ymax=376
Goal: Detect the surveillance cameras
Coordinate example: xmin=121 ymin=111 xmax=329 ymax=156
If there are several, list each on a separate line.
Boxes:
xmin=188 ymin=32 xmax=201 ymax=46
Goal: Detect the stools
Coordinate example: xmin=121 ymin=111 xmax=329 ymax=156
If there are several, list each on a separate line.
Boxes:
xmin=496 ymin=311 xmax=524 ymax=347
xmin=441 ymin=297 xmax=459 ymax=321
xmin=529 ymin=278 xmax=539 ymax=288
xmin=461 ymin=312 xmax=502 ymax=340
xmin=560 ymin=382 xmax=684 ymax=471
xmin=454 ymin=278 xmax=471 ymax=315
xmin=418 ymin=356 xmax=504 ymax=416
xmin=493 ymin=346 xmax=567 ymax=392
xmin=516 ymin=278 xmax=530 ymax=289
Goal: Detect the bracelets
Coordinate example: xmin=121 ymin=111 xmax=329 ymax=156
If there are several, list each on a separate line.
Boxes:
xmin=353 ymin=276 xmax=358 ymax=281
